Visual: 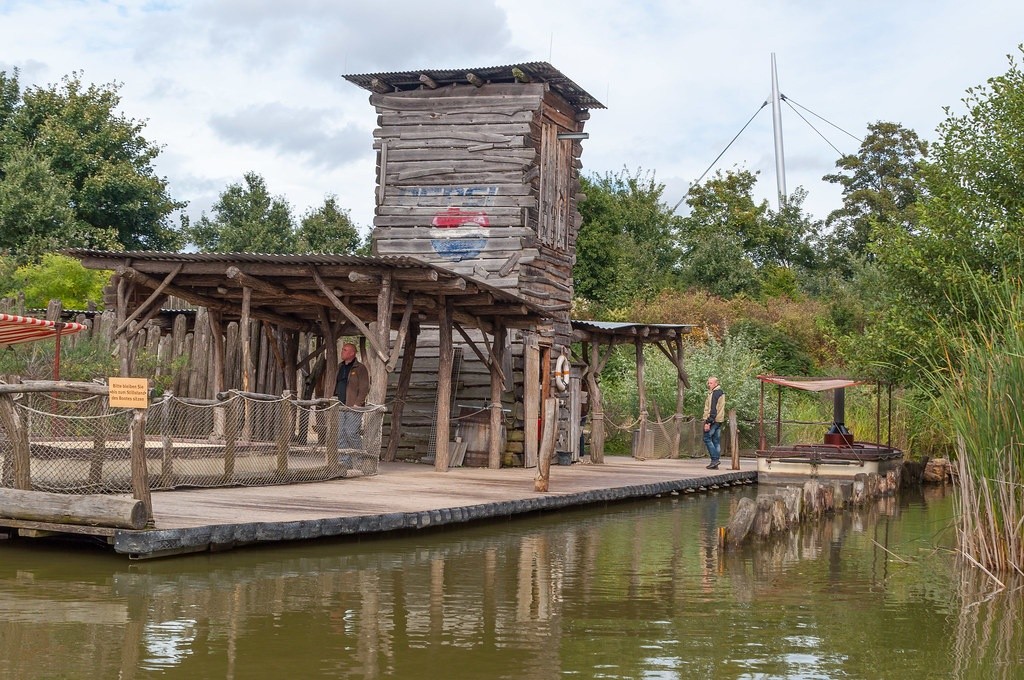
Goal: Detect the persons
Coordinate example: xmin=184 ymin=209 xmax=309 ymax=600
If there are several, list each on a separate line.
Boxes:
xmin=334 ymin=343 xmax=369 ymax=464
xmin=702 ymin=376 xmax=725 ymax=469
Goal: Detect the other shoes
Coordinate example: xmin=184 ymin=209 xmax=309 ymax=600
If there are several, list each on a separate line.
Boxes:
xmin=706 ymin=460 xmax=721 ymax=470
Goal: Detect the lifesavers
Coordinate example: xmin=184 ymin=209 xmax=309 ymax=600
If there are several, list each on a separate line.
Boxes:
xmin=555 ymin=354 xmax=570 ymax=392
